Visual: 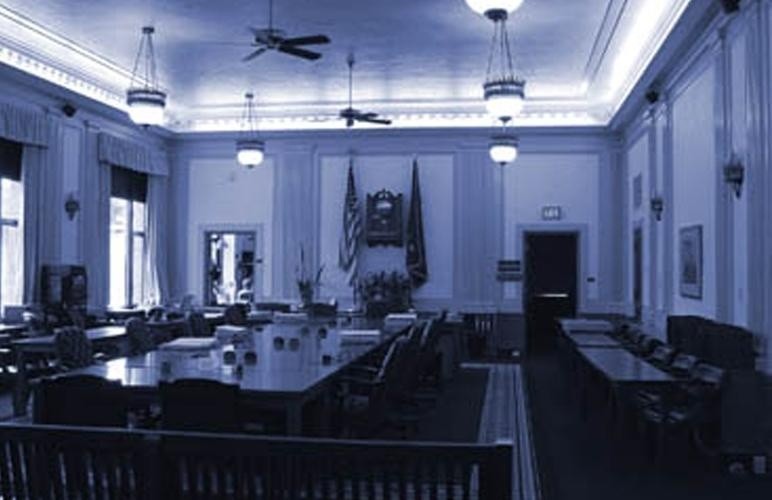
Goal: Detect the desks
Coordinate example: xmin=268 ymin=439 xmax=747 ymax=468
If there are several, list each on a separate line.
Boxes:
xmin=555 ymin=316 xmax=679 ymax=441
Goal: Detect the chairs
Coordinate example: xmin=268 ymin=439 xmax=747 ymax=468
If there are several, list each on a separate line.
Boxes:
xmin=606 ymin=315 xmax=727 ymax=451
xmin=0 ymin=294 xmax=457 ymax=436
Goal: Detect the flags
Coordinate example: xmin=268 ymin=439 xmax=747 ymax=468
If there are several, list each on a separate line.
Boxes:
xmin=405 ymin=156 xmax=429 ymax=286
xmin=338 ymin=163 xmax=362 ymax=288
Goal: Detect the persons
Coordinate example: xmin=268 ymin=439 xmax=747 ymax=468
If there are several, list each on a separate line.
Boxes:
xmin=237 ymin=277 xmax=254 ymax=301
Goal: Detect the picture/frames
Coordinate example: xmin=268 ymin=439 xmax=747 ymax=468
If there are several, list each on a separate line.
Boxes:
xmin=678 ymin=223 xmax=706 ymax=301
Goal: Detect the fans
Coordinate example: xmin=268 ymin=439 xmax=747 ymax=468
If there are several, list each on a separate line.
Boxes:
xmin=237 ymin=0 xmax=392 ymax=130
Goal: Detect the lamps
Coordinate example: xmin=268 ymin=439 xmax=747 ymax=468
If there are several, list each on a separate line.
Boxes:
xmin=124 ymin=25 xmax=168 ymax=132
xmin=478 ymin=7 xmax=527 ymax=167
xmin=232 ymin=91 xmax=266 ymax=171
